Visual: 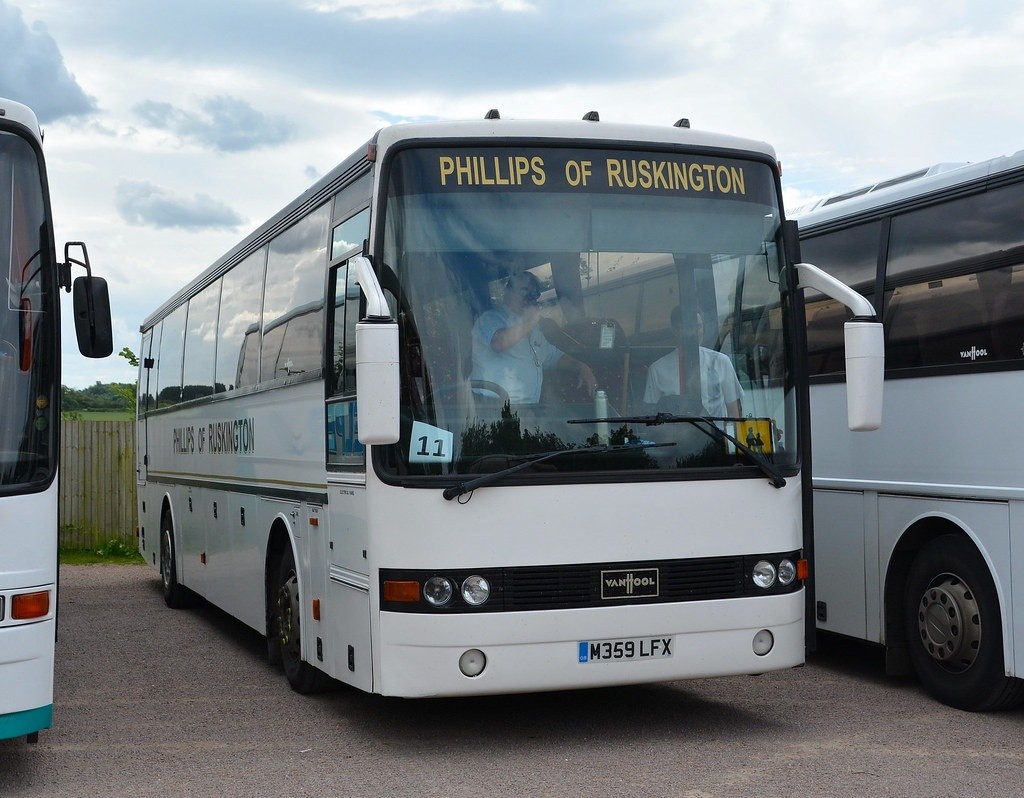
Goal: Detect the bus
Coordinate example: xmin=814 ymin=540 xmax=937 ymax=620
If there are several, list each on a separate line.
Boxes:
xmin=800 ymin=265 xmax=1023 ymax=354
xmin=0 ymin=95 xmax=114 ymax=743
xmin=543 ymin=149 xmax=1024 ymax=712
xmin=231 ymin=285 xmax=365 ymax=467
xmin=135 ymin=110 xmax=885 ymax=700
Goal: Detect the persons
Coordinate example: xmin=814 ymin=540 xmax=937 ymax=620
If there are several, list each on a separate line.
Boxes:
xmin=644 ymin=303 xmax=745 ymax=419
xmin=467 ymin=270 xmax=598 ymax=405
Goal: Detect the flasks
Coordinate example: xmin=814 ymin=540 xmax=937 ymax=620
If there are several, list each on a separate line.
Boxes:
xmin=594 ymin=389 xmax=610 ymax=447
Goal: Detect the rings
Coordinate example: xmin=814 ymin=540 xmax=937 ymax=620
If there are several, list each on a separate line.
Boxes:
xmin=592 ymin=383 xmax=597 ymax=386
